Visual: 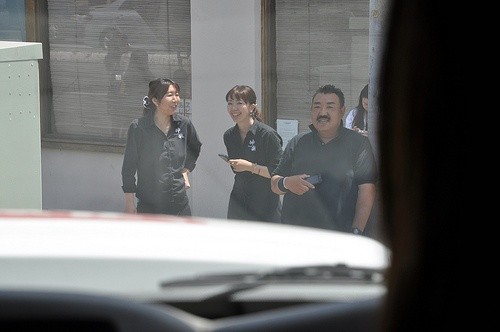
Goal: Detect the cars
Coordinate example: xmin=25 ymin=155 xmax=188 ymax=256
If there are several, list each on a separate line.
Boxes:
xmin=82 ymin=1 xmax=173 ymax=51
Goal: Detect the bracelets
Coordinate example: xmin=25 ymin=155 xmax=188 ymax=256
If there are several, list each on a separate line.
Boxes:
xmin=251 ymin=162 xmax=261 ymax=175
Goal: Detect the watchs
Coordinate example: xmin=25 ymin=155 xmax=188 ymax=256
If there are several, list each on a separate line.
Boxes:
xmin=351 ymin=227 xmax=363 ymax=235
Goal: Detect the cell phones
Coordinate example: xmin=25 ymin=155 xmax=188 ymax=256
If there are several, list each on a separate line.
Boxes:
xmin=218 ymin=153 xmax=230 ymax=162
xmin=304 ymin=174 xmax=322 ymax=185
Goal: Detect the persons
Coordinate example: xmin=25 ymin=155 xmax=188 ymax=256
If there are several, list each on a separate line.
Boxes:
xmin=121 ymin=78 xmax=202 ymax=216
xmin=223 ymin=85 xmax=283 ymax=225
xmin=104 ymin=32 xmax=153 ymax=142
xmin=271 ymin=84 xmax=376 ymax=239
xmin=345 ymin=84 xmax=368 ymax=137
